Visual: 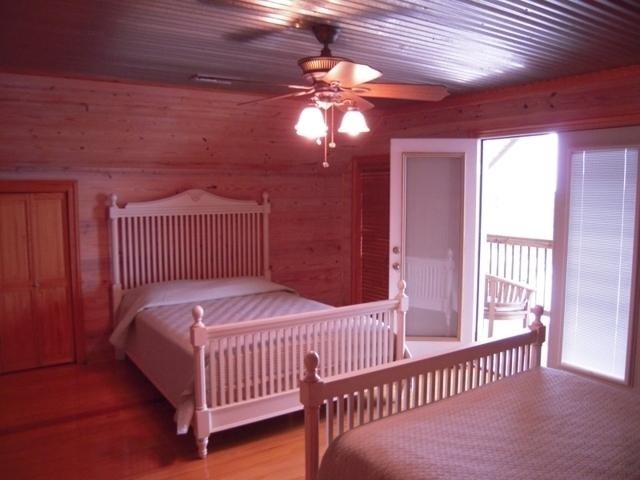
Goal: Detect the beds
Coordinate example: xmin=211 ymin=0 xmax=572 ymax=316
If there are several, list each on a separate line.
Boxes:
xmin=107 ymin=188 xmax=411 ymax=461
xmin=299 ymin=302 xmax=640 ymax=479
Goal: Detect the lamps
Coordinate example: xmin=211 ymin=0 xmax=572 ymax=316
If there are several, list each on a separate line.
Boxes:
xmin=291 ymin=86 xmax=372 ymax=169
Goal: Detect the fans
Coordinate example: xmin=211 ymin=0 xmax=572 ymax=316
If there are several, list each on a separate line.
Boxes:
xmin=234 ymin=22 xmax=451 ymax=115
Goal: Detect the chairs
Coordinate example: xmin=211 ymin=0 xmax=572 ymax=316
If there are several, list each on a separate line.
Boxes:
xmin=484 ymin=276 xmax=535 ymax=338
